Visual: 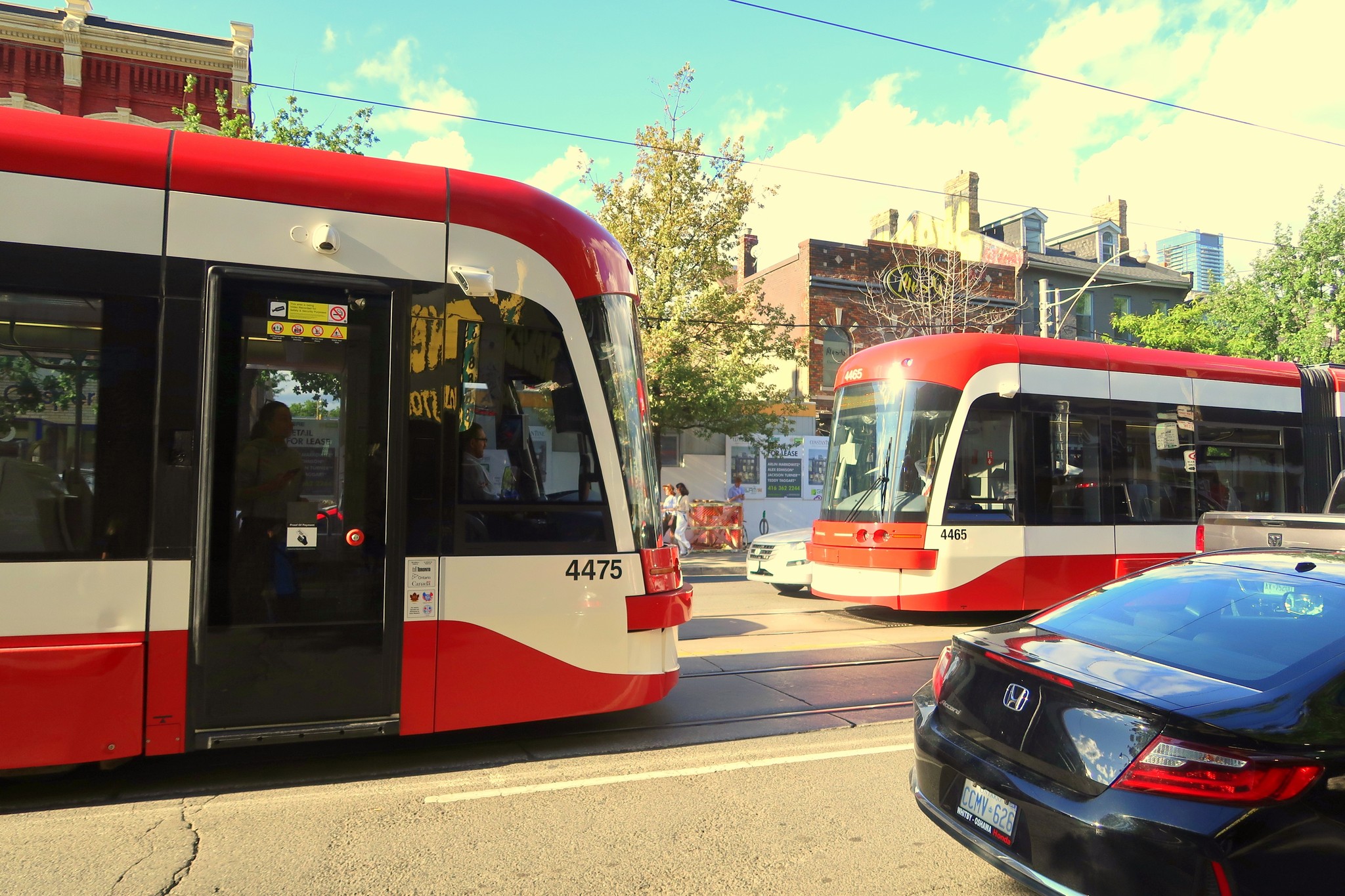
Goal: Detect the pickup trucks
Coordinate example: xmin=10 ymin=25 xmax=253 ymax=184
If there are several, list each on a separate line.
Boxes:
xmin=1194 ymin=476 xmax=1344 ymax=596
xmin=1044 ymin=477 xmax=1226 ymax=528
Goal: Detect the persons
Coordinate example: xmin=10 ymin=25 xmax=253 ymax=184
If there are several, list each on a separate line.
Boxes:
xmin=914 ymin=457 xmax=935 ymax=497
xmin=459 ymin=422 xmax=515 ymax=503
xmin=1198 ymin=461 xmax=1242 ymax=513
xmin=661 ymin=483 xmax=694 ymax=557
xmin=727 ymin=477 xmax=746 ymax=545
xmin=234 ymin=401 xmax=317 ymax=622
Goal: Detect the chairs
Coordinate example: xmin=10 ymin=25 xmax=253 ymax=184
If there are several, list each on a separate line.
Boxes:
xmin=1103 ymin=481 xmax=1222 ymax=525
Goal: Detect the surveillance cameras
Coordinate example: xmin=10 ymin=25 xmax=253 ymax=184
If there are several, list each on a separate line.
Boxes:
xmin=454 ymin=270 xmax=494 ymax=293
xmin=316 ymin=227 xmax=336 ymax=250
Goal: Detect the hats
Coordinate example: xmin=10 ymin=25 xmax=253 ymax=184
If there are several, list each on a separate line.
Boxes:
xmin=663 ymin=484 xmax=674 ymax=490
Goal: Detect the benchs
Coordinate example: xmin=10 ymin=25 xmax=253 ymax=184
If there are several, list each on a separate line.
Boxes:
xmin=1135 ymin=607 xmax=1315 ymax=667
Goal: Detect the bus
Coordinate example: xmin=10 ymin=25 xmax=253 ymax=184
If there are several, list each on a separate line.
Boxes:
xmin=807 ymin=338 xmax=1344 ymax=611
xmin=0 ymin=106 xmax=692 ymax=775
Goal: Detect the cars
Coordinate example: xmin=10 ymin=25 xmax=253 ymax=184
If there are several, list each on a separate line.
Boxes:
xmin=746 ymin=487 xmax=928 ymax=593
xmin=911 ymin=548 xmax=1344 ymax=895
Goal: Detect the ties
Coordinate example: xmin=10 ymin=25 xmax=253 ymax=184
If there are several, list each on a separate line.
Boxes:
xmin=923 ymin=484 xmax=932 ymax=496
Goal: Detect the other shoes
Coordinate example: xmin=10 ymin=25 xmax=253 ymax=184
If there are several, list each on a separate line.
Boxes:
xmin=686 ymin=546 xmax=693 ymax=556
xmin=678 ymin=554 xmax=686 ymax=557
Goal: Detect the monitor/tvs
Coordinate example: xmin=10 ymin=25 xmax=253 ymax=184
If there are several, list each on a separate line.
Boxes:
xmin=502 ymin=414 xmax=529 ymax=450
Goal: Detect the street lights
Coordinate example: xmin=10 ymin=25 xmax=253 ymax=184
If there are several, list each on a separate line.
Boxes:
xmin=1038 ymin=244 xmax=1148 ymax=339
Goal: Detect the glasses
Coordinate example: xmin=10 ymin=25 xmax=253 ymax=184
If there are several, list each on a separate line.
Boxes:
xmin=260 ymin=399 xmax=275 ymax=423
xmin=475 ymin=438 xmax=488 ymax=442
